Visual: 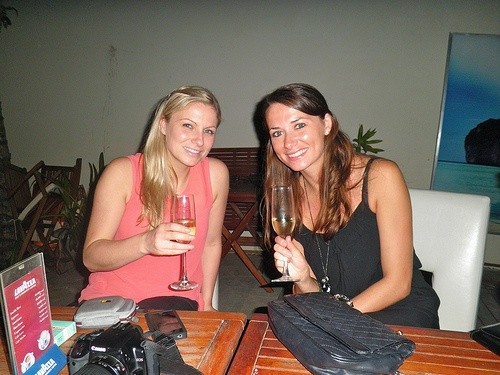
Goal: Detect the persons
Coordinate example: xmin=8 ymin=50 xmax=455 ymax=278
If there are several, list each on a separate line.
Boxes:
xmin=71 ymin=86 xmax=229 ymax=311
xmin=259 ymin=83 xmax=441 ymax=329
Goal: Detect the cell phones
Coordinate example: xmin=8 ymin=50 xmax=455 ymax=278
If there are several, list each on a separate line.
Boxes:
xmin=144 ymin=310 xmax=187 ymax=340
xmin=73 ymin=295 xmax=136 ymax=327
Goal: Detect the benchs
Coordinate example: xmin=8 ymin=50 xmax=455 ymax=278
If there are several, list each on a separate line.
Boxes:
xmin=207 ymin=148 xmax=275 ymax=246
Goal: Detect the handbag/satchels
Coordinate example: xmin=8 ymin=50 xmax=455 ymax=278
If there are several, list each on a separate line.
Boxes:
xmin=266 ymin=292 xmax=416 ymax=375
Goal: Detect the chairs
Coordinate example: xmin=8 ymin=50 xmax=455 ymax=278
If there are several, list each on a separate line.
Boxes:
xmin=32 ymin=158 xmax=82 ymax=260
xmin=408 ymin=190 xmax=490 ymax=333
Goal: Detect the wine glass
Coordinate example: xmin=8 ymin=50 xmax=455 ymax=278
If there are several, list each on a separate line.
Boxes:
xmin=169 ymin=194 xmax=199 ymax=290
xmin=271 ymin=185 xmax=300 ymax=282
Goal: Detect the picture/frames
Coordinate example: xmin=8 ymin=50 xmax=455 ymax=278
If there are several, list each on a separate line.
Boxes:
xmin=429 ymin=31 xmax=500 ymax=236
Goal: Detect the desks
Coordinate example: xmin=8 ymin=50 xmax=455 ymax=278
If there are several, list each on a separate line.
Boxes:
xmin=228 ymin=314 xmax=500 ymax=375
xmin=222 ymin=184 xmax=274 ymax=294
xmin=0 ymin=307 xmax=247 ymax=375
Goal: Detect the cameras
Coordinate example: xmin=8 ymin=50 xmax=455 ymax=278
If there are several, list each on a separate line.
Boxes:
xmin=66 ymin=323 xmax=205 ymax=375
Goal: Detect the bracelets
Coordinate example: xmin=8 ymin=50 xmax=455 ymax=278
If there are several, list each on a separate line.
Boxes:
xmin=333 ymin=294 xmax=354 ymax=307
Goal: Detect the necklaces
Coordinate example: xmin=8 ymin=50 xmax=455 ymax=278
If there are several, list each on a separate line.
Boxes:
xmin=302 ymin=177 xmax=331 ymax=294
